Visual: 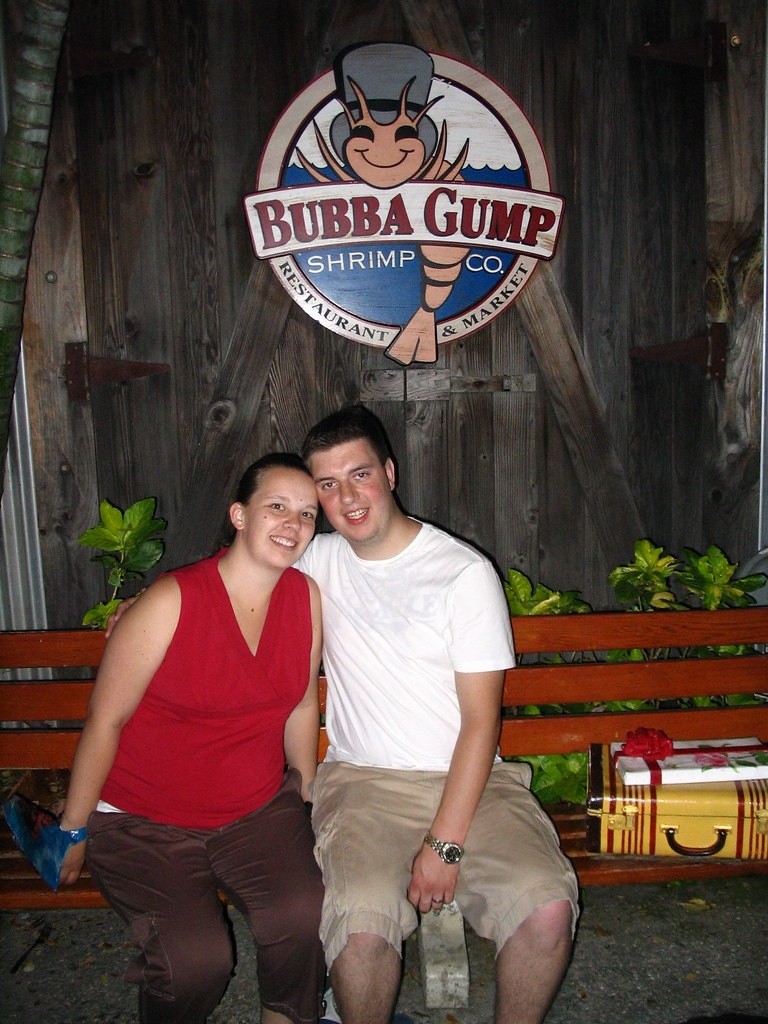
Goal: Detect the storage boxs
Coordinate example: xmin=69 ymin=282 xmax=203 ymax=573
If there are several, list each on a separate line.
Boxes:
xmin=610 ymin=726 xmax=768 ymax=786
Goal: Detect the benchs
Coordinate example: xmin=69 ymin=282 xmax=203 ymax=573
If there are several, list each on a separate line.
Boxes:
xmin=0 ymin=605 xmax=768 ymax=918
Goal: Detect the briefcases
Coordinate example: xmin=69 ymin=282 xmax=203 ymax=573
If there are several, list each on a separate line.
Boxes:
xmin=586 ymin=742 xmax=768 ymax=860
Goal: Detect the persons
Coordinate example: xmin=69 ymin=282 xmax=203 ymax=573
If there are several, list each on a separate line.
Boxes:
xmin=43 ymin=454 xmax=326 ymax=1024
xmin=106 ymin=406 xmax=580 ymax=1024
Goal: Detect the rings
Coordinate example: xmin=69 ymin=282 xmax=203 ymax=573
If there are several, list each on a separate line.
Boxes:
xmin=432 ymin=898 xmax=443 ymax=903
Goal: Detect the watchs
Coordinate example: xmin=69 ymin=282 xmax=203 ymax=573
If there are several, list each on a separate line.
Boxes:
xmin=424 ymin=832 xmax=464 ymax=864
